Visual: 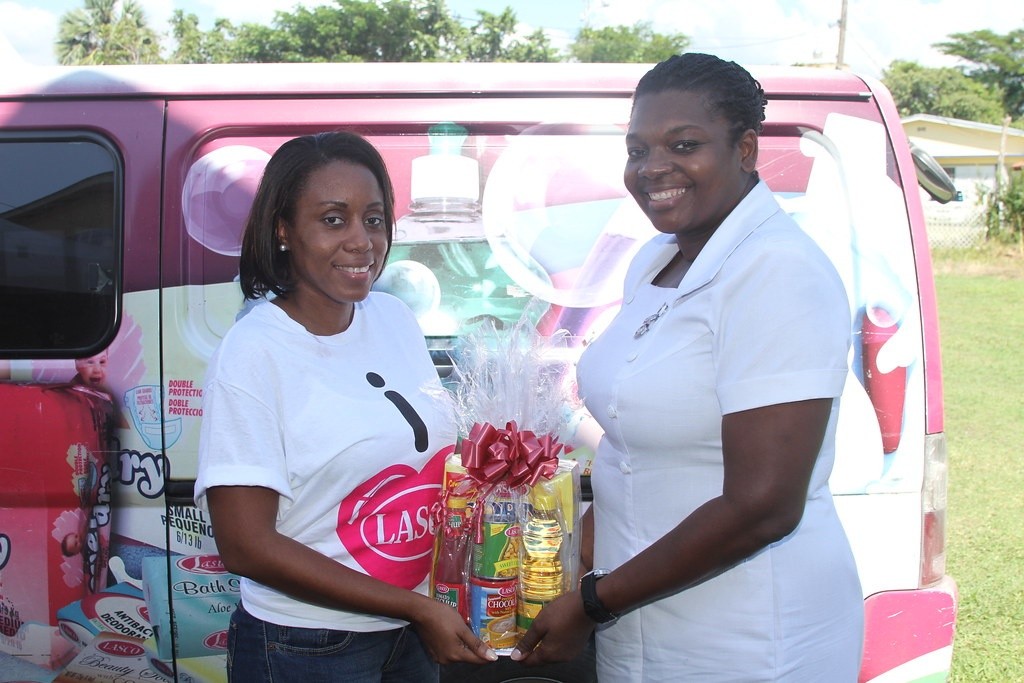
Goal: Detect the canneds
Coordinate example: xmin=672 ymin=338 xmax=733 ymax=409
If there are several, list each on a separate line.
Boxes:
xmin=464 ymin=515 xmax=522 ymax=649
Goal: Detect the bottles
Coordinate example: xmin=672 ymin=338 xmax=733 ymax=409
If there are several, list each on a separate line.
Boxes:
xmin=434 ymin=496 xmax=469 ymax=628
xmin=517 ymin=494 xmax=565 ymax=649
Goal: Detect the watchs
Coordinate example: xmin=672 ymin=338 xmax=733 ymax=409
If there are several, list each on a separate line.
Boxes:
xmin=580 ymin=569 xmax=622 ymax=626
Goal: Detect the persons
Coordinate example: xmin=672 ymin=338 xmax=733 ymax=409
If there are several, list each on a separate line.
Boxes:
xmin=62 ymin=516 xmax=90 ymax=571
xmin=510 ymin=52 xmax=864 ymax=683
xmin=193 ymin=131 xmax=499 ymax=683
xmin=67 ymin=349 xmax=131 ymax=429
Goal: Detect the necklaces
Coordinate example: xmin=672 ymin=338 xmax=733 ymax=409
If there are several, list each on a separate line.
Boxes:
xmin=632 ymin=260 xmax=686 ymax=339
